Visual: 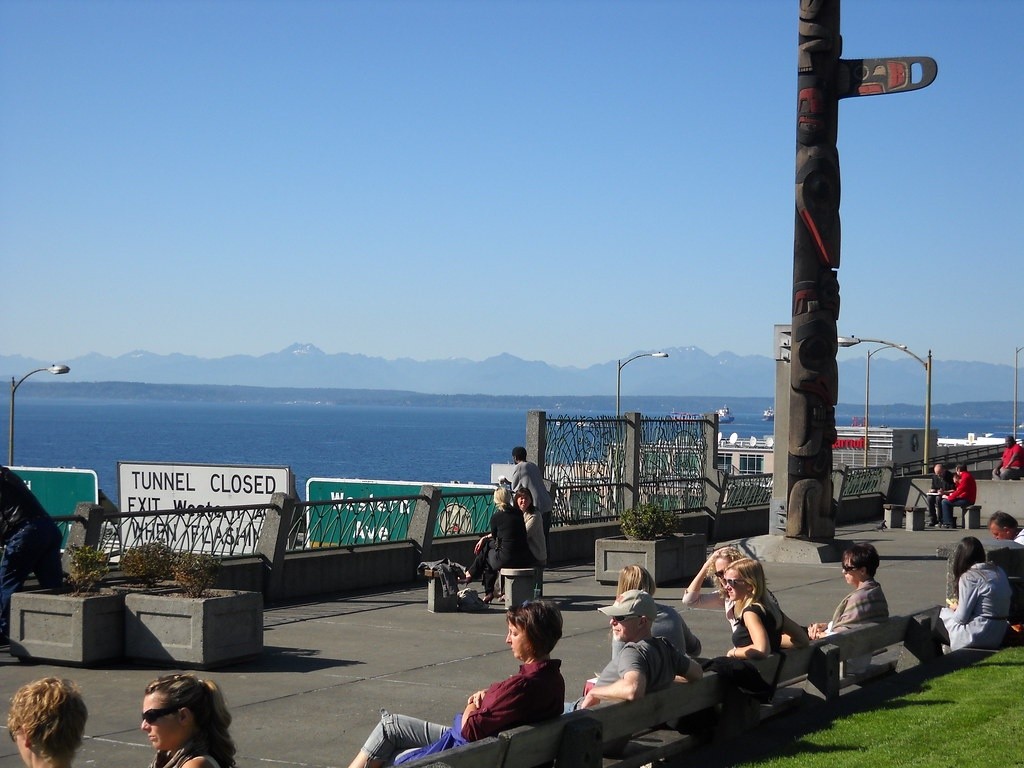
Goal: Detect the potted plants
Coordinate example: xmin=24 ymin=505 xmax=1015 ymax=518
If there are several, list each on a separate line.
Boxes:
xmin=10 ymin=541 xmax=264 ymax=664
xmin=594 ymin=503 xmax=707 ymax=583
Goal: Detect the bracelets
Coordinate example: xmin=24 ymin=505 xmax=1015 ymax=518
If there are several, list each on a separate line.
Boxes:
xmin=485 ymin=535 xmax=489 ymax=539
xmin=733 ymin=648 xmax=739 ymax=657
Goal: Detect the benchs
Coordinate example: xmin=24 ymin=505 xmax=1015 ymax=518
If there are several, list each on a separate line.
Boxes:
xmin=363 ymin=599 xmax=1024 ymax=768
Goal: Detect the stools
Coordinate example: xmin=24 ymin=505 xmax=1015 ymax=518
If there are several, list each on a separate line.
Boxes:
xmin=425 ymin=569 xmax=462 ymax=613
xmin=961 ymin=505 xmax=982 ymax=529
xmin=500 ymin=567 xmax=538 ymax=609
xmin=884 ymin=503 xmax=904 ymax=529
xmin=905 ymin=506 xmax=926 ymax=530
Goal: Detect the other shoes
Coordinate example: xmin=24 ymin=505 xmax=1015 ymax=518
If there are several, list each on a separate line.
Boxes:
xmin=929 ymin=522 xmax=941 ymax=529
xmin=498 ymin=595 xmax=505 ymax=602
xmin=941 ymin=524 xmax=957 ymax=529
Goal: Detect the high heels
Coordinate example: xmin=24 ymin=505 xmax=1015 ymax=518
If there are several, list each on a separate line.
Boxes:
xmin=482 ymin=593 xmax=495 ymax=605
xmin=457 ymin=577 xmax=473 ymax=587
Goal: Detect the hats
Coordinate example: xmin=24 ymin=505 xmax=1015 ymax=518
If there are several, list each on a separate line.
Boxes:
xmin=597 ymin=590 xmax=657 ymax=621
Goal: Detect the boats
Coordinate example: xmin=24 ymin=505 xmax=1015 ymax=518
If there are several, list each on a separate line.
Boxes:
xmin=668 ymin=403 xmax=735 ymax=424
xmin=763 ymin=406 xmax=774 ymax=421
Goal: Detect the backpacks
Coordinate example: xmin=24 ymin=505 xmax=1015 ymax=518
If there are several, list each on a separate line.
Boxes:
xmin=457 ymin=588 xmax=489 ymax=611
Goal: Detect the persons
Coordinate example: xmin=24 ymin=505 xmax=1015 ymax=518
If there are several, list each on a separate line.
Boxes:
xmin=142 ymin=675 xmax=239 ymax=768
xmin=0 ymin=465 xmax=64 ymax=641
xmin=560 ymin=436 xmax=1024 ymax=759
xmin=456 ymin=447 xmax=552 ymax=604
xmin=5 ymin=678 xmax=88 ymax=768
xmin=349 ymin=600 xmax=565 ymax=768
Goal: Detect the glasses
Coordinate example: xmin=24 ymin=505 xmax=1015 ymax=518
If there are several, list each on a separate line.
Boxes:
xmin=141 ymin=707 xmax=176 ymax=724
xmin=715 ymin=570 xmax=724 ymax=578
xmin=841 ymin=563 xmax=856 ymax=572
xmin=612 ymin=616 xmax=638 ymax=622
xmin=10 ymin=726 xmax=20 ymax=742
xmin=721 ymin=578 xmax=742 ymax=587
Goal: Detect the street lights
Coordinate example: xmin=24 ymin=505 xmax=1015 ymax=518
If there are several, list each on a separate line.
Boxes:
xmin=615 ymin=353 xmax=669 ymax=416
xmin=8 ymin=365 xmax=71 ymax=466
xmin=837 ymin=336 xmax=932 ymax=476
